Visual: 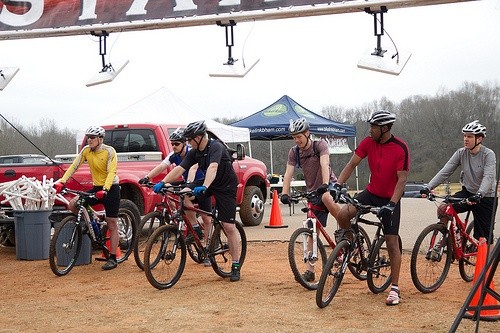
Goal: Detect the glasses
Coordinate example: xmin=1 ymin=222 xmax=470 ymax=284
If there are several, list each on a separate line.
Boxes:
xmin=87 ymin=135 xmax=99 ymax=139
xmin=171 ymin=143 xmax=181 ymax=146
xmin=188 ymin=137 xmax=196 ymax=142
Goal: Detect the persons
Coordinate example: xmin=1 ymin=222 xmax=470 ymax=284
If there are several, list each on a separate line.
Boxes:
xmin=138 ymin=131 xmax=211 ymax=266
xmin=420 ymin=123 xmax=497 ymax=260
xmin=329 ymin=111 xmax=410 ymax=304
xmin=281 ymin=121 xmax=338 ymax=282
xmin=56 ymin=126 xmax=121 ymax=269
xmin=153 ymin=120 xmax=240 ymax=281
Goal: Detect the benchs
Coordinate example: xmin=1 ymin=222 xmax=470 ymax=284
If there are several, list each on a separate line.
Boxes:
xmin=126 ymin=141 xmax=154 ymax=151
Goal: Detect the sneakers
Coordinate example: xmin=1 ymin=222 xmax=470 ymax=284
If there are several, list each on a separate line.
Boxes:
xmin=185 ymin=227 xmax=201 ymax=242
xmin=102 ymin=259 xmax=117 ymax=269
xmin=385 ymin=291 xmax=399 ymax=305
xmin=231 ymin=264 xmax=241 ymax=281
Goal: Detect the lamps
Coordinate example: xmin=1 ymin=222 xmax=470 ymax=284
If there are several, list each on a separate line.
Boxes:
xmin=0 ymin=65 xmax=19 ymax=90
xmin=209 ymin=19 xmax=261 ymax=78
xmin=85 ymin=29 xmax=130 ymax=87
xmin=357 ymin=5 xmax=412 ymax=75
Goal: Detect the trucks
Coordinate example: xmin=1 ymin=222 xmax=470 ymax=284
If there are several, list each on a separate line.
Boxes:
xmin=54 ymin=143 xmax=87 ymax=162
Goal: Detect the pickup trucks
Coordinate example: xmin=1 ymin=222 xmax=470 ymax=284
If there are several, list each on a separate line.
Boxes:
xmin=0 ymin=121 xmax=271 ymax=227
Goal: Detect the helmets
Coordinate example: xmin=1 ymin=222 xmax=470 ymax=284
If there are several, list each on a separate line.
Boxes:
xmin=85 ymin=125 xmax=105 ymax=136
xmin=184 ymin=121 xmax=206 ymax=138
xmin=288 ymin=118 xmax=309 ymax=134
xmin=168 ymin=129 xmax=185 ymax=141
xmin=462 ymin=121 xmax=486 ymax=137
xmin=367 ymin=110 xmax=397 ymax=126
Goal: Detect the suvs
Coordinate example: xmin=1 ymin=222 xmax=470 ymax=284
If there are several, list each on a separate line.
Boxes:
xmin=403 ymin=184 xmax=424 ymax=198
xmin=0 ymin=154 xmax=53 ymax=164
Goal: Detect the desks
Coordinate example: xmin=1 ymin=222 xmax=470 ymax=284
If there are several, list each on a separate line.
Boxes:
xmin=267 ymin=181 xmax=307 ymax=216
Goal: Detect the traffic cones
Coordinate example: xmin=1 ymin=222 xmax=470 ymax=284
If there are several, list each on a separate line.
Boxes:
xmin=264 ymin=189 xmax=289 ymax=228
xmin=463 ymin=236 xmax=500 ymax=321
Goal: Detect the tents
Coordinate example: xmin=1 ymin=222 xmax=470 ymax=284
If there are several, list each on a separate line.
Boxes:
xmin=205 ymin=120 xmax=252 ymax=158
xmin=227 ymin=95 xmax=359 ymax=191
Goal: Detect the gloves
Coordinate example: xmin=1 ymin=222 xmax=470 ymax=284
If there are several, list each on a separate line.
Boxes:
xmin=96 ymin=190 xmax=107 ymax=198
xmin=153 ymin=181 xmax=164 ymax=193
xmin=193 ymin=185 xmax=206 ymax=196
xmin=55 ymin=182 xmax=65 ymax=195
xmin=316 ymin=184 xmax=329 ymax=194
xmin=280 ymin=194 xmax=291 ymax=204
xmin=378 ymin=202 xmax=396 ymax=219
xmin=330 ymin=183 xmax=342 ymax=203
xmin=138 ymin=176 xmax=150 ymax=184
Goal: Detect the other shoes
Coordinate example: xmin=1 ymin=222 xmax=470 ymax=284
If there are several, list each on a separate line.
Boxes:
xmin=439 ymin=237 xmax=447 ymax=247
xmin=302 ymin=270 xmax=315 ymax=281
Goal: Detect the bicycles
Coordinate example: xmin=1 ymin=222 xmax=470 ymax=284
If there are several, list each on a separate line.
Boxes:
xmin=315 ymin=187 xmax=403 ymax=306
xmin=282 ymin=184 xmax=371 ymax=290
xmin=132 ymin=181 xmax=247 ymax=289
xmin=409 ymin=189 xmax=480 ymax=293
xmin=49 ymin=184 xmax=137 ymax=276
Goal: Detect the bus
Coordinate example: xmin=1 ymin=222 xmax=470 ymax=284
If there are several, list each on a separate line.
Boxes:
xmin=268 ymin=175 xmax=307 ymax=197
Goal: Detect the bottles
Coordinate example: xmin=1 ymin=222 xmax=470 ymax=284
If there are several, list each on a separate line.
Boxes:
xmin=92 ymin=222 xmax=99 ymax=234
